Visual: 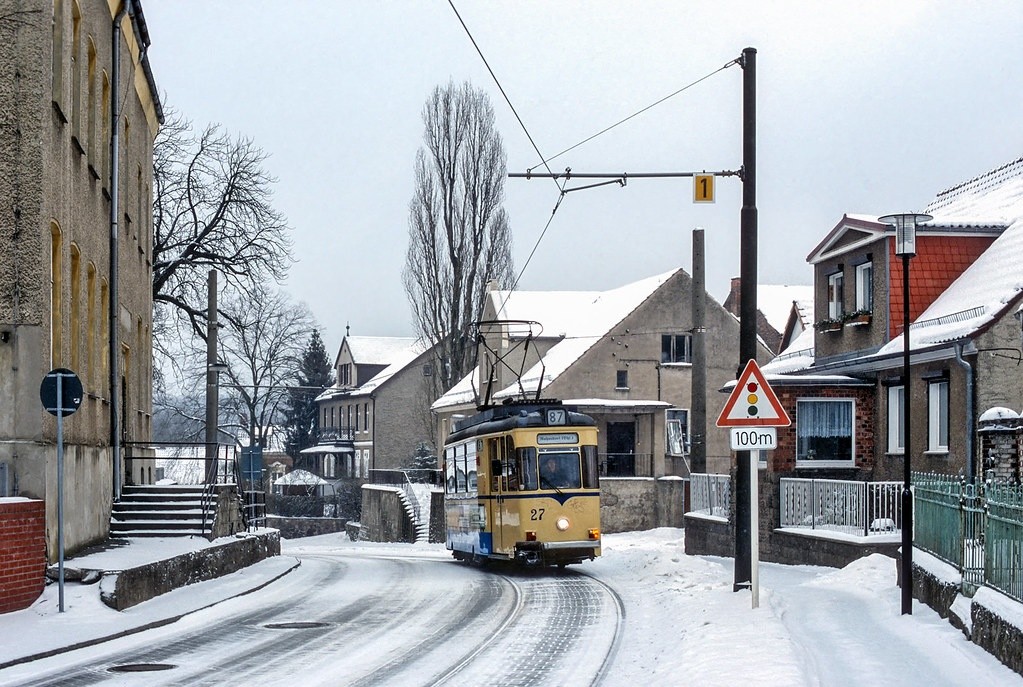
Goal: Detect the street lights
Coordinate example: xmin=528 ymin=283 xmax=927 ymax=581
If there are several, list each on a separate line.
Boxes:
xmin=204 ymin=361 xmax=229 ymax=484
xmin=877 ymin=213 xmax=932 ymax=616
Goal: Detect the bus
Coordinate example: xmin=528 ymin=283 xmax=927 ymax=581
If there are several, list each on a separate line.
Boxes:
xmin=444 ymin=319 xmax=603 ymax=571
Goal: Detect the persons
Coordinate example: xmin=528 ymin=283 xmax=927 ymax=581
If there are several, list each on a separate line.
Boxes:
xmin=540 ymin=457 xmax=570 ymax=489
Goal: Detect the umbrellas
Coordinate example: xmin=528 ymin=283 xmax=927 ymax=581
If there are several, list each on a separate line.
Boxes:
xmin=273 ymin=469 xmax=331 ymax=485
